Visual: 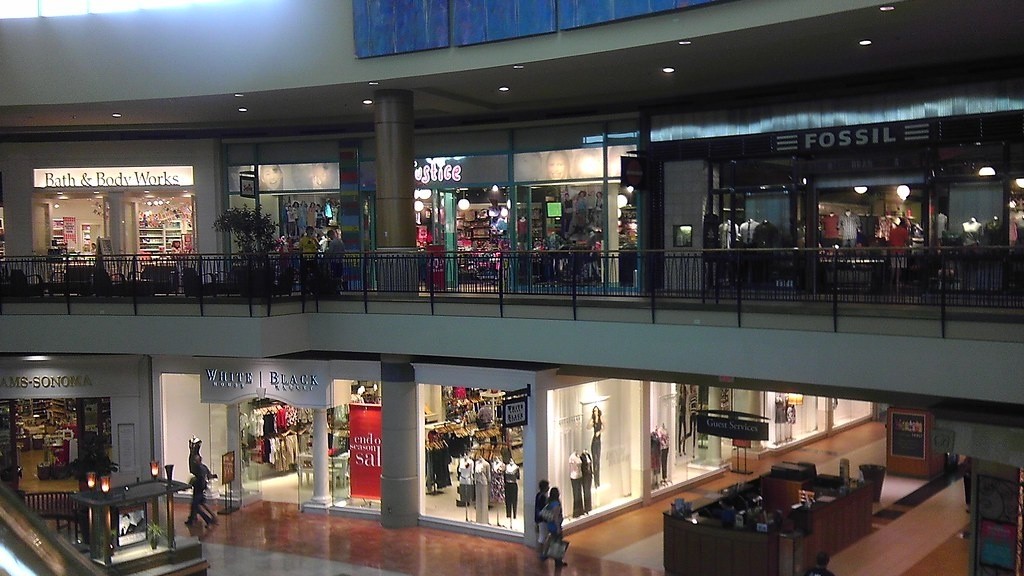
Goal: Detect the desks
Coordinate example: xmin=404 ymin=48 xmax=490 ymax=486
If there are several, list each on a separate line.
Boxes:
xmin=300 ymin=452 xmax=349 ymax=488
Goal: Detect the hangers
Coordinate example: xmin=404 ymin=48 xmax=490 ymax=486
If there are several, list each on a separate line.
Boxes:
xmin=250 ymin=401 xmax=350 ymax=442
xmin=425 ymin=397 xmax=509 ymax=460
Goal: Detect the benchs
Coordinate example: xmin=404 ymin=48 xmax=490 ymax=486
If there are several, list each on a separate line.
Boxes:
xmin=302 ymin=466 xmax=344 ymax=490
xmin=91 ymin=269 xmax=127 ymax=298
xmin=22 ymin=490 xmax=82 ymax=541
xmin=127 ymin=266 xmax=180 ymax=297
xmin=182 ymin=265 xmax=235 ymax=297
xmin=46 ymin=264 xmax=96 ymax=296
xmin=0 ymin=268 xmax=45 ymax=298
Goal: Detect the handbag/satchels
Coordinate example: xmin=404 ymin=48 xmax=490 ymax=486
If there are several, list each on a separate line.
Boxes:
xmin=539 ymin=503 xmax=556 ymax=522
xmin=548 ymin=540 xmax=569 ymax=558
xmin=537 ymin=523 xmax=548 ymax=543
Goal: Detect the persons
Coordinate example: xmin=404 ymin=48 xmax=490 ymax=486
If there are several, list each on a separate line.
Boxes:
xmin=478 ymin=401 xmax=492 ymax=427
xmin=535 ymin=480 xmax=567 ymax=568
xmin=1010 ymin=210 xmax=1024 ymax=247
xmin=564 ymin=191 xmax=603 ymax=236
xmin=805 ymin=551 xmax=836 ymax=576
xmin=171 ymin=240 xmax=182 ymax=285
xmin=586 ymin=405 xmax=605 ymax=489
xmin=189 ymin=435 xmax=202 ymax=472
xmin=460 ymin=451 xmax=520 ymax=519
xmin=569 ymin=449 xmax=593 ymax=518
xmin=650 ymin=422 xmax=670 ymax=489
xmin=185 ymin=454 xmax=219 ymax=529
xmin=90 ymin=243 xmax=96 ymax=253
xmin=287 ymin=201 xmax=326 ymax=239
xmin=887 ymin=217 xmax=909 ymax=288
xmin=718 ymin=219 xmax=778 ymax=289
xmin=822 ymin=210 xmax=911 ymax=248
xmin=961 ymin=217 xmax=1004 ymax=246
xmin=121 ymin=510 xmax=145 ymax=536
xmin=299 ymin=226 xmax=345 ymax=296
xmin=679 ymin=384 xmax=698 ymax=459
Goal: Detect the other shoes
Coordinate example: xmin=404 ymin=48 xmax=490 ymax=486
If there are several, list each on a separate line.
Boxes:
xmin=560 ymin=562 xmax=567 ymax=566
xmin=185 ymin=518 xmax=196 ymax=524
xmin=205 ymin=520 xmax=212 ymax=528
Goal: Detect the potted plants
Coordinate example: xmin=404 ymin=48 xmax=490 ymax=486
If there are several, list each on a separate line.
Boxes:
xmin=209 ymin=203 xmax=286 ymax=298
xmin=100 ymin=528 xmax=118 ymax=557
xmin=146 ymin=518 xmax=165 ymax=550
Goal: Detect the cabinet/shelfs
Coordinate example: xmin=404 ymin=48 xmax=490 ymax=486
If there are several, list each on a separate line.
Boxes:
xmin=52 ymin=219 xmax=64 ymax=238
xmin=138 ymin=219 xmax=183 ymax=254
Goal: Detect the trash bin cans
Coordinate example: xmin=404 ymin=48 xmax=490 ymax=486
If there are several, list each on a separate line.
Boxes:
xmin=859 ymin=464 xmax=886 ymax=502
